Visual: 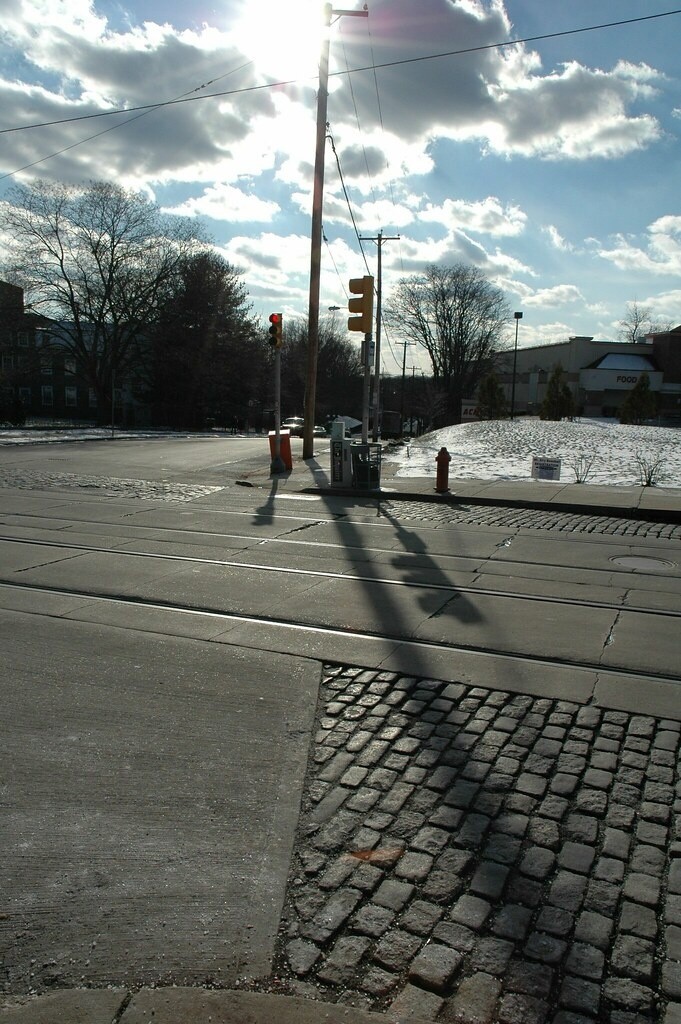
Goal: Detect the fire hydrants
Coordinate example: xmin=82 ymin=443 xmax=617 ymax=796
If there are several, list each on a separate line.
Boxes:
xmin=433 ymin=447 xmax=452 ymax=494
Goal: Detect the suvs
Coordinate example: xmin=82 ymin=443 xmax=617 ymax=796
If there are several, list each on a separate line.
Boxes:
xmin=282 ymin=417 xmax=304 ymax=436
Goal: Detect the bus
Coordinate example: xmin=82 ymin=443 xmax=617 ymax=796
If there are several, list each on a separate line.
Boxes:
xmin=381 ymin=410 xmax=401 ymax=440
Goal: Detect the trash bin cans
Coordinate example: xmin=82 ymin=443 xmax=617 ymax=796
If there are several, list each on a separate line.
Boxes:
xmin=350 ymin=442 xmax=382 ymax=491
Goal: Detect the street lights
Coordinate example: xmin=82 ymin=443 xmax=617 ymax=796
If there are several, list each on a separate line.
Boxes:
xmin=510 ymin=311 xmax=523 ymax=419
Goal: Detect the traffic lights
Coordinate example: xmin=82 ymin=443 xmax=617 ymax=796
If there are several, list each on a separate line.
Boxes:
xmin=348 ymin=276 xmax=374 ymax=334
xmin=268 ymin=313 xmax=282 ymax=348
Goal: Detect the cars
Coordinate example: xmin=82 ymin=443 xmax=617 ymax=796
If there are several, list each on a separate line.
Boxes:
xmin=344 ymin=427 xmax=350 ymax=437
xmin=313 ymin=426 xmax=327 ymax=438
